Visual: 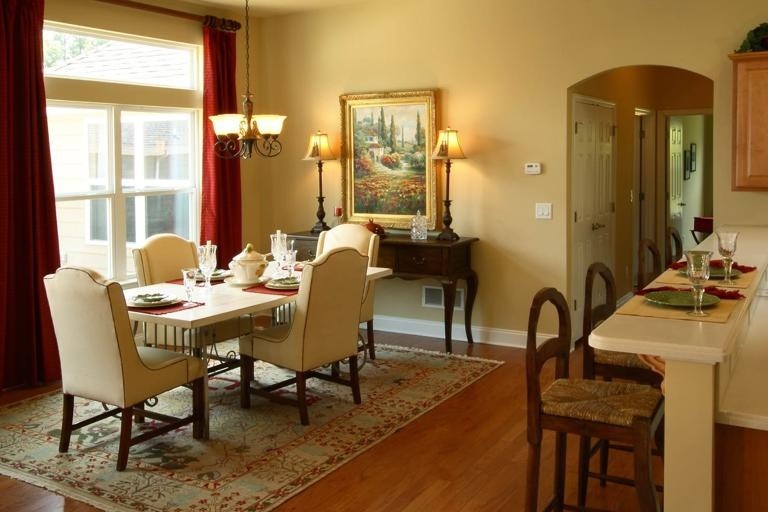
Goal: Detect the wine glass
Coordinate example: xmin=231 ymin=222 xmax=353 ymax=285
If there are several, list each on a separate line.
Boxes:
xmin=197 ymin=245 xmax=217 ymax=294
xmin=270 ymin=233 xmax=287 ymax=280
xmin=682 ymin=250 xmax=714 ymax=321
xmin=714 ymin=231 xmax=740 ymax=286
xmin=182 ymin=268 xmax=199 ymax=307
xmin=285 ymin=249 xmax=298 ymax=280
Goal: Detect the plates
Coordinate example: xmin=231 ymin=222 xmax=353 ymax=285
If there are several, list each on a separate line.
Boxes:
xmin=264 ymin=277 xmax=301 ymax=289
xmin=223 ymin=277 xmax=272 ymax=286
xmin=272 ymin=271 xmax=303 ymax=281
xmin=126 ymin=298 xmax=181 ymax=307
xmin=138 ymin=294 xmax=167 ymax=302
xmin=680 ymin=266 xmax=742 ymax=280
xmin=187 ymin=268 xmax=232 ymax=282
xmin=644 ymin=289 xmax=720 ymax=308
xmin=131 ymin=296 xmax=177 ymax=304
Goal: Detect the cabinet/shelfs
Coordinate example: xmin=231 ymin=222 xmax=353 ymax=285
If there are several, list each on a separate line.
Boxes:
xmin=729 ymin=52 xmax=767 ymax=192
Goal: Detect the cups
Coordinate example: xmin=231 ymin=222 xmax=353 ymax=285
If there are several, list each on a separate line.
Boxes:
xmin=334 ymin=206 xmax=343 ymax=225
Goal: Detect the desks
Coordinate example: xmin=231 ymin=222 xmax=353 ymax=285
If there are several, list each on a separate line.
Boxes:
xmin=287 ymin=230 xmax=480 ymax=355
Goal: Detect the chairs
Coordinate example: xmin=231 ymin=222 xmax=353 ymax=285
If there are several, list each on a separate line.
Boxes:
xmin=43 ymin=266 xmax=208 ymax=471
xmin=578 ymin=262 xmax=665 ymax=512
xmin=638 ymin=239 xmax=661 ymax=291
xmin=239 ymin=247 xmax=369 ymax=426
xmin=665 ymin=227 xmax=682 ymax=269
xmin=305 ymin=224 xmax=380 ymax=376
xmin=126 ymin=233 xmax=254 ymax=391
xmin=525 ymin=286 xmax=665 ymax=512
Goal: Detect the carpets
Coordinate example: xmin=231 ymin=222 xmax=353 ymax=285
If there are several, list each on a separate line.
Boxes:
xmin=1 ymin=328 xmax=505 ymax=512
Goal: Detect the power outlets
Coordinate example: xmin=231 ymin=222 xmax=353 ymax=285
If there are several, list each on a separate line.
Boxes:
xmin=525 ymin=163 xmax=541 ymax=175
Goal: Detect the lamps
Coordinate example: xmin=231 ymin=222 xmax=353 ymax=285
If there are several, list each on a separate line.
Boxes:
xmin=301 ymin=130 xmax=337 ymax=233
xmin=209 ymin=0 xmax=288 ymax=159
xmin=430 ymin=126 xmax=467 ymax=241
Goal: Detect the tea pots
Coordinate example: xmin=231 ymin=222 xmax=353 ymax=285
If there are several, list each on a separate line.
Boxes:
xmin=228 ymin=242 xmax=268 ymax=282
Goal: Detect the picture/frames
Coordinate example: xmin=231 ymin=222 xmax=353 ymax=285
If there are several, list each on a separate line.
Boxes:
xmin=684 ymin=150 xmax=690 ymax=180
xmin=691 ymin=143 xmax=696 ymax=172
xmin=338 ymin=88 xmax=440 ymax=230
